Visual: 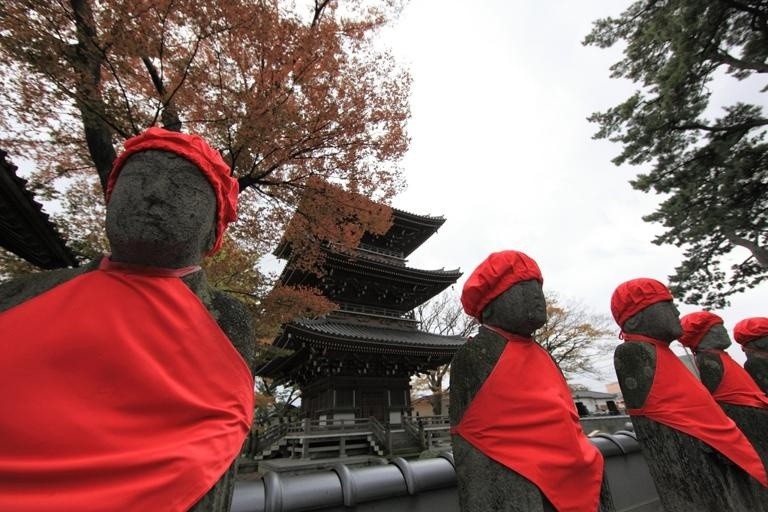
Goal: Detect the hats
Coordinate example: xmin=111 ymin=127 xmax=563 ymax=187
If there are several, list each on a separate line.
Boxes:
xmin=734 ymin=317 xmax=768 ymax=346
xmin=611 ymin=278 xmax=673 ymax=331
xmin=678 ymin=311 xmax=723 ymax=352
xmin=106 ymin=126 xmax=240 ymax=257
xmin=461 ymin=250 xmax=543 ymax=324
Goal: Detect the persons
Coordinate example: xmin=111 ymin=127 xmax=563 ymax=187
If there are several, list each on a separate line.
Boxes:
xmin=732 ymin=316 xmax=768 ymax=398
xmin=670 ymin=312 xmax=768 ymax=478
xmin=1 ymin=126 xmax=262 ymax=512
xmin=446 ymin=248 xmax=616 ymax=511
xmin=602 ymin=277 xmax=767 ymax=492
